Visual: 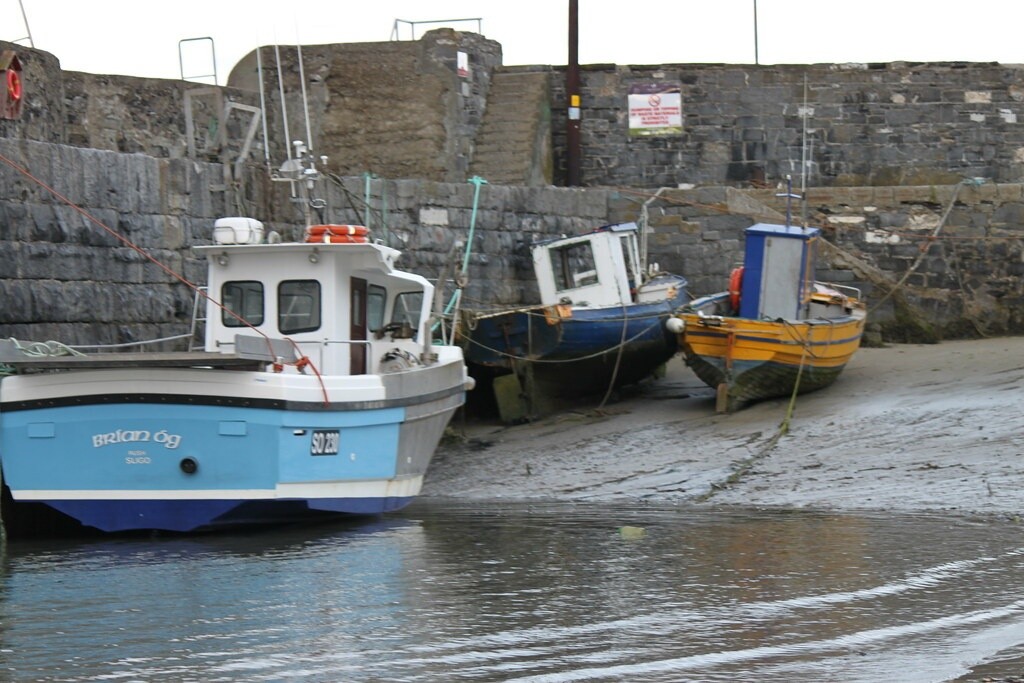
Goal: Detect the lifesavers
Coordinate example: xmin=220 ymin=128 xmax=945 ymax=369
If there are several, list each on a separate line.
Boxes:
xmin=729 ymin=266 xmax=744 ymax=310
xmin=303 ymin=224 xmax=370 ymax=243
xmin=7 ymin=69 xmax=20 ymax=100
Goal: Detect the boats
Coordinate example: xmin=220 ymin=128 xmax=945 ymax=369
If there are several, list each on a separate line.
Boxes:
xmin=664 ymin=66 xmax=868 ymax=415
xmin=0 ymin=28 xmax=477 ymax=543
xmin=424 ymin=181 xmax=696 ymax=432
